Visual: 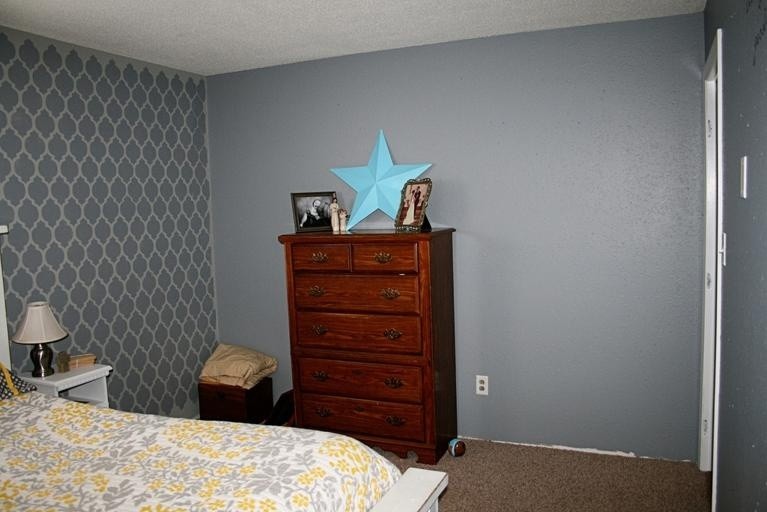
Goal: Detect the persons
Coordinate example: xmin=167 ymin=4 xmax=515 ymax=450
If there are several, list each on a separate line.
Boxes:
xmin=338 ymin=208 xmax=348 ymax=232
xmin=300 ymin=198 xmax=322 ymax=227
xmin=329 ymin=197 xmax=340 ymax=232
xmin=414 ymin=185 xmax=422 ymax=219
xmin=403 ymin=189 xmax=415 ymax=225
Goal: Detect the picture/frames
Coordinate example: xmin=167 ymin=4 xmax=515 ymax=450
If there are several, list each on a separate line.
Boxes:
xmin=290 ymin=191 xmax=337 ymax=233
xmin=394 ymin=178 xmax=433 ymax=233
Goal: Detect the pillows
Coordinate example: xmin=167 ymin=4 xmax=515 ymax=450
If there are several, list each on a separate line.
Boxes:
xmin=0 ymin=362 xmax=36 ymax=402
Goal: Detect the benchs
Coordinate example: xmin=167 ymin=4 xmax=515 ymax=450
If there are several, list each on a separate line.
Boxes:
xmin=368 ymin=466 xmax=449 ymax=512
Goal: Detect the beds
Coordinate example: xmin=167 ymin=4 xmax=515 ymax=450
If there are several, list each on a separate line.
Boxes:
xmin=0 ymin=225 xmax=402 ymax=512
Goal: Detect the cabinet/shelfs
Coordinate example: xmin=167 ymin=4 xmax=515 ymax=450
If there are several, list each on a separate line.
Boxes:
xmin=278 ymin=228 xmax=457 ymax=464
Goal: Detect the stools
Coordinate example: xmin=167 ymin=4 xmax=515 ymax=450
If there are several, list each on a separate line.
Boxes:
xmin=197 ymin=376 xmax=273 ymax=425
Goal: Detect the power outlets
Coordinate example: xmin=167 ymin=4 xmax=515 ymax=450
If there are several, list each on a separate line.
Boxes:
xmin=475 ymin=375 xmax=488 ymax=395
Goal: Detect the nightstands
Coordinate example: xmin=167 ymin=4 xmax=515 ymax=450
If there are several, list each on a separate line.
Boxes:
xmin=17 ymin=364 xmax=114 ymax=408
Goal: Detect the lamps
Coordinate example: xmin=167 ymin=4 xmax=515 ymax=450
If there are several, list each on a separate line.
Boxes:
xmin=12 ymin=301 xmax=69 ymax=377
